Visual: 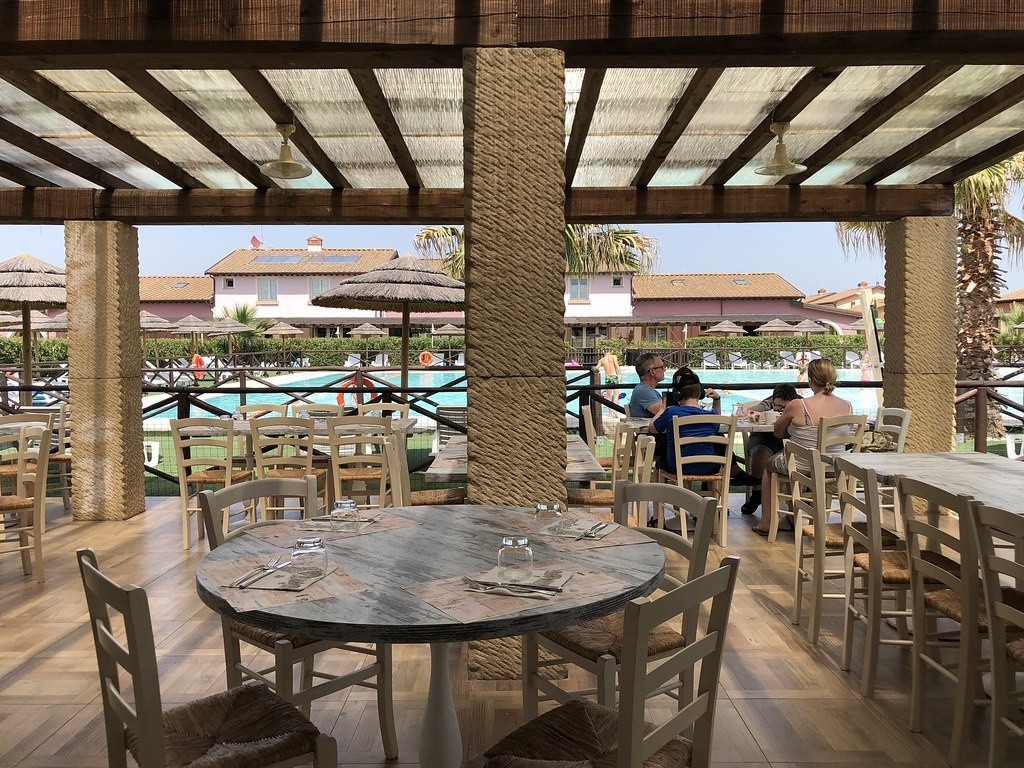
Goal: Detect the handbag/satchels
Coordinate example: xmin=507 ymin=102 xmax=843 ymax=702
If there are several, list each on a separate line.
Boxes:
xmin=853 ymin=432 xmax=898 ymax=453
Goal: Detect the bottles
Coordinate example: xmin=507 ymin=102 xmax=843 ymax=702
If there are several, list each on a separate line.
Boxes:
xmin=748 ymin=411 xmax=756 ymax=423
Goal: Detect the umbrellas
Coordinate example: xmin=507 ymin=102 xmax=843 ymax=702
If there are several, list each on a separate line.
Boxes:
xmin=260 ymin=321 xmax=305 ymax=360
xmin=209 ymin=314 xmax=255 ymax=366
xmin=793 ymin=317 xmax=831 ymax=346
xmin=310 ymin=252 xmax=465 ymax=418
xmin=346 ymin=323 xmax=388 ymax=367
xmin=137 ymin=309 xmax=178 ymax=369
xmin=171 ymin=314 xmax=227 ymax=356
xmin=0 ymin=253 xmax=66 ymax=406
xmin=753 ymin=317 xmax=800 ymax=369
xmin=429 ymin=323 xmax=465 ymax=366
xmin=704 ymin=320 xmax=748 ymax=370
xmin=845 ymin=318 xmax=885 ymax=348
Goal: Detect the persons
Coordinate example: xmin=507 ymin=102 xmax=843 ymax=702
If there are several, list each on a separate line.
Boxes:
xmin=647 ymin=374 xmax=722 ymax=538
xmin=595 ymin=345 xmax=623 ymax=418
xmin=629 ymin=352 xmax=763 ymax=485
xmin=740 ymin=382 xmax=805 ymax=515
xmin=751 ymin=357 xmax=854 ymax=536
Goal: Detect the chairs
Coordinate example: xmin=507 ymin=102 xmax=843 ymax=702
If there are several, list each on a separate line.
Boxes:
xmin=344 ymin=354 xmax=362 ymax=368
xmin=841 ymin=351 xmax=884 ymax=369
xmin=701 ymin=352 xmax=720 ymax=370
xmin=780 ymin=350 xmax=822 ymax=369
xmin=76 ymin=403 xmax=1024 ymax=768
xmin=141 ymin=356 xmax=310 ymax=387
xmin=371 ymin=354 xmax=390 ymax=367
xmin=0 ymin=404 xmax=71 ymax=584
xmin=727 ymin=352 xmax=750 ymax=370
xmin=430 ymin=354 xmax=444 ymax=367
xmin=454 ymin=354 xmax=463 ymax=366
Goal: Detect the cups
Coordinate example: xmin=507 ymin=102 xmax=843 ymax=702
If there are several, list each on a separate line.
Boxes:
xmin=533 ymin=503 xmax=564 ymax=535
xmin=765 ymin=409 xmax=783 ymax=424
xmin=329 ymin=499 xmax=360 ymax=532
xmin=292 ymin=537 xmax=327 ymax=582
xmin=497 ymin=536 xmax=534 ymax=581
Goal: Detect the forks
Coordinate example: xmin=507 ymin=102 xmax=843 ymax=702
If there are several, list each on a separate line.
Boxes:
xmin=461 ymin=576 xmax=565 ymax=596
xmin=320 ymin=512 xmax=380 ymax=520
xmin=221 ymin=553 xmax=283 ymax=589
xmin=585 ymin=523 xmax=609 ymax=539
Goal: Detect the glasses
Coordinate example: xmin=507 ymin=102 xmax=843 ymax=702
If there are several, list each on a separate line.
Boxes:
xmin=773 ymin=403 xmax=786 ymax=410
xmin=647 ymin=364 xmax=664 ymax=373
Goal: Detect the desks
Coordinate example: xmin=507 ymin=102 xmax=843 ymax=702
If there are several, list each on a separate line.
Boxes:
xmin=179 ymin=419 xmax=420 ymax=530
xmin=423 ymin=434 xmax=607 ymax=483
xmin=194 ymin=504 xmax=665 ymax=767
xmin=619 ymin=416 xmax=869 ymax=531
xmin=820 ymin=451 xmax=1024 ymax=712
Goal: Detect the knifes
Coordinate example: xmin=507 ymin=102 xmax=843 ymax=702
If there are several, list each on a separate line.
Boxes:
xmin=574 ymin=520 xmax=602 ymax=542
xmin=311 ymin=519 xmax=375 ymax=522
xmin=471 ymin=580 xmax=563 ymax=592
xmin=239 ymin=561 xmax=291 ymax=589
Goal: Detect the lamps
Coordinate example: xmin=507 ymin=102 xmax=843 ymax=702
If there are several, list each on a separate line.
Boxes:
xmin=260 ymin=124 xmax=313 ymax=179
xmin=754 ymin=122 xmax=808 ymax=176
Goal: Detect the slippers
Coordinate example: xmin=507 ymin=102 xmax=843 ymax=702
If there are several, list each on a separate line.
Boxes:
xmin=751 ymin=523 xmax=769 ymax=535
xmin=778 ymin=515 xmax=795 ymax=532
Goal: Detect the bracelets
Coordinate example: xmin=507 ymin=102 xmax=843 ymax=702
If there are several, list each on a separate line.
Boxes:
xmin=713 ymin=396 xmax=720 ymax=401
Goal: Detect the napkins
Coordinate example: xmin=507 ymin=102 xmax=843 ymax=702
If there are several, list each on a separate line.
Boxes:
xmin=538 ymin=518 xmax=620 ymax=540
xmin=463 ymin=566 xmax=573 ymax=599
xmin=221 ymin=563 xmax=339 ymax=591
xmin=295 ymin=517 xmax=378 ymax=533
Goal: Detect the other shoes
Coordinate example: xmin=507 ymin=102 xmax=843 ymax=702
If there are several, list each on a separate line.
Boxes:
xmin=650 ymin=516 xmax=667 ymax=530
xmin=673 ymin=505 xmax=689 ymax=519
xmin=730 ymin=471 xmax=762 ymax=487
xmin=741 ymin=489 xmax=761 ymax=514
